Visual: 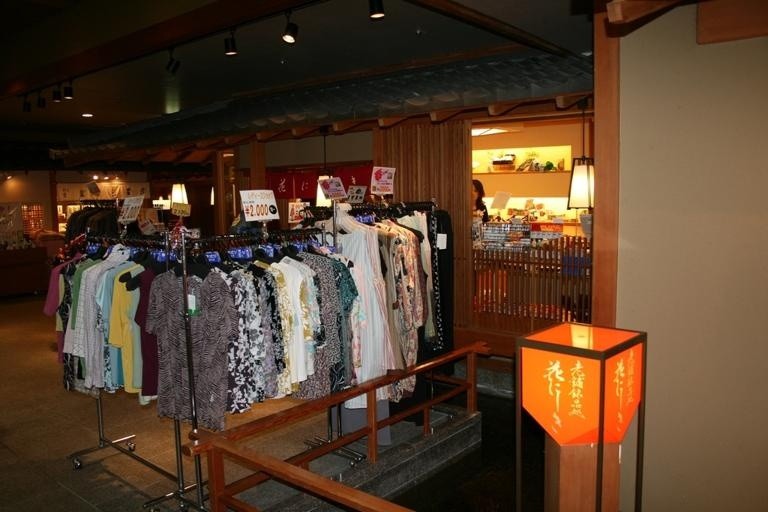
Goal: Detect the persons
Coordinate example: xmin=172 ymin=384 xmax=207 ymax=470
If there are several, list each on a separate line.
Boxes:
xmin=472 ymin=179 xmax=489 ymax=223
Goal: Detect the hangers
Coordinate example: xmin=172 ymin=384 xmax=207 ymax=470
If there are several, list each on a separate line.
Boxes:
xmin=50 ymin=196 xmax=437 ymax=291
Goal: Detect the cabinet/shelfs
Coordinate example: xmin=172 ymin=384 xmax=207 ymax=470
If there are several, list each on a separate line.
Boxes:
xmin=0 ymin=246 xmax=48 ymax=300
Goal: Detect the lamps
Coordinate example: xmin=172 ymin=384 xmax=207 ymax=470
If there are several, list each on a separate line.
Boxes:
xmin=164 ymin=0 xmax=388 ymax=73
xmin=514 ymin=319 xmax=648 ymax=512
xmin=567 ymin=98 xmax=595 ymax=211
xmin=20 ymin=78 xmax=75 ymax=114
